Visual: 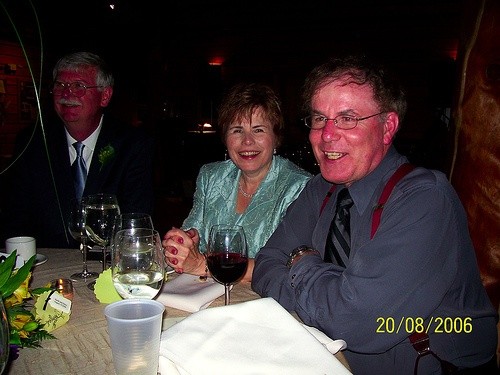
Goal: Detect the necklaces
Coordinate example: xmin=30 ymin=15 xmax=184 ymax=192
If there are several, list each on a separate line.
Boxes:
xmin=237 ymin=184 xmax=258 ymax=199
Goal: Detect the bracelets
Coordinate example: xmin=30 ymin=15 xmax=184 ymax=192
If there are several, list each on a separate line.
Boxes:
xmin=198 ymin=255 xmax=211 ymax=283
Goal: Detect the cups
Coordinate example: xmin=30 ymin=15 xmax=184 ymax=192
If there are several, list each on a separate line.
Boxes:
xmin=103 ymin=298 xmax=165 ymax=375
xmin=6 ymin=236 xmax=36 ymax=260
xmin=49 ymin=278 xmax=73 ymax=295
xmin=112 ymin=212 xmax=155 ymax=246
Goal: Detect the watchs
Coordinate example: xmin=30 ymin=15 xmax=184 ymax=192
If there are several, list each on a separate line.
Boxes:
xmin=284 ymin=246 xmax=318 ymax=269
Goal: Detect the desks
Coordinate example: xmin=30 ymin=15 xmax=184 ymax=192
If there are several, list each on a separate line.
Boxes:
xmin=7 ymin=249 xmax=351 ymax=375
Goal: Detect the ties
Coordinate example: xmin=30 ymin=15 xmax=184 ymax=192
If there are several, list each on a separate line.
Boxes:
xmin=71 ymin=141 xmax=88 ymax=206
xmin=325 ymin=188 xmax=353 ymax=270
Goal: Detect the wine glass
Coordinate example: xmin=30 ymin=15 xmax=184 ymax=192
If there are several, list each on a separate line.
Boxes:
xmin=111 ymin=228 xmax=166 ymax=298
xmin=84 ymin=193 xmax=122 ymax=290
xmin=206 ymin=223 xmax=249 ymax=305
xmin=67 ymin=197 xmax=100 ymax=281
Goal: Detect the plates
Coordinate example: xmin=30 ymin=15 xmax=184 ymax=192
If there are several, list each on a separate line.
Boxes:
xmin=31 ymin=254 xmax=48 ymax=267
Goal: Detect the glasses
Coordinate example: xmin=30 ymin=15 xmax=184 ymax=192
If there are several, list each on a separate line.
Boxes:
xmin=49 ymin=81 xmax=105 ymax=99
xmin=301 ymin=110 xmax=387 ymax=130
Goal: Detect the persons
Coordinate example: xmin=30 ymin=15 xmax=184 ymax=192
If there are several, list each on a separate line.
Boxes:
xmin=163 ymin=84 xmax=312 ymax=281
xmin=0 ymin=53 xmax=157 ymax=248
xmin=253 ymin=54 xmax=500 ymax=375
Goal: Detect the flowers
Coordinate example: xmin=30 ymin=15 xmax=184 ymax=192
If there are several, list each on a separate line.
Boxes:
xmin=0 ymin=249 xmax=59 ymax=351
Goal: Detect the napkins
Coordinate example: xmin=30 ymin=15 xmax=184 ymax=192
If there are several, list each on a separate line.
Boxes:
xmin=157 ymin=297 xmax=354 ymax=375
xmin=156 ymin=272 xmax=234 ymax=313
